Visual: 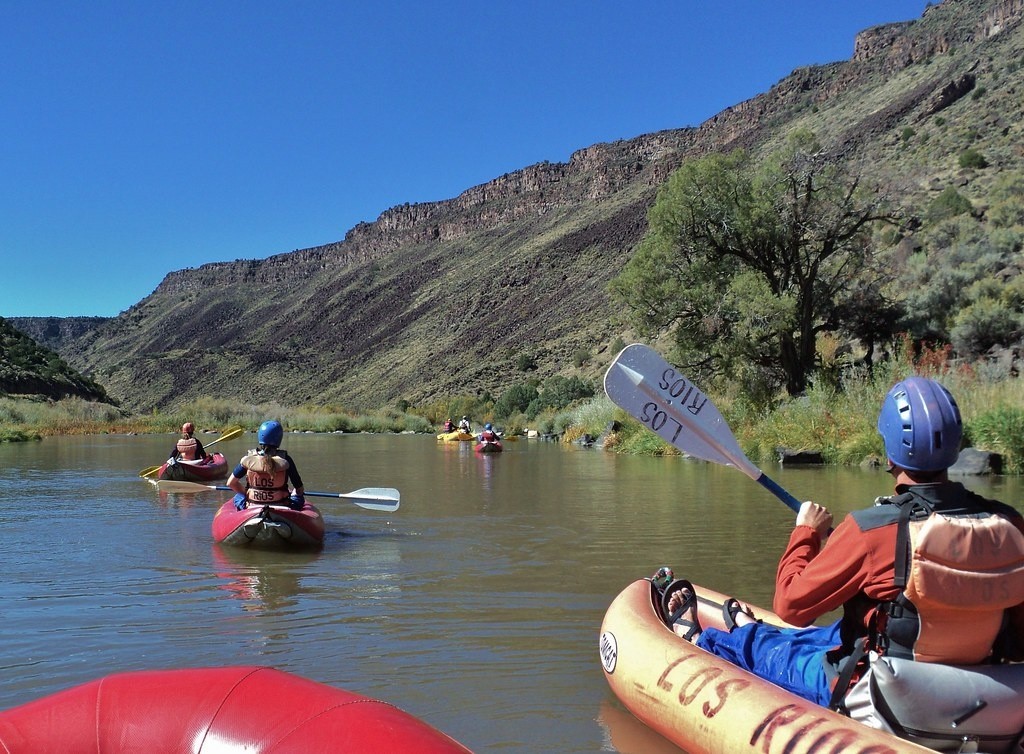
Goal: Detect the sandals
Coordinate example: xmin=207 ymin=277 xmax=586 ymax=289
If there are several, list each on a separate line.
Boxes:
xmin=722 ymin=597 xmax=748 ymax=632
xmin=661 ymin=579 xmax=702 ymax=642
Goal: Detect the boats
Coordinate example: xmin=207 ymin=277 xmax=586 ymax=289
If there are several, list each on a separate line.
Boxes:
xmin=158 ymin=451 xmax=228 ymax=480
xmin=598 ymin=567 xmax=1024 ymax=754
xmin=0 ymin=663 xmax=476 ymax=754
xmin=504 ymin=436 xmax=519 ymax=442
xmin=212 ymin=491 xmax=325 ymax=552
xmin=474 ymin=438 xmax=502 ymax=453
xmin=436 ymin=432 xmax=474 ymax=441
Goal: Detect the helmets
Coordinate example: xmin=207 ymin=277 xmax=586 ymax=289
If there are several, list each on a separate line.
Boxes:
xmin=183 ymin=422 xmax=194 ymax=433
xmin=877 ymin=376 xmax=963 ymax=472
xmin=258 ymin=420 xmax=283 ymax=446
xmin=463 ymin=416 xmax=466 ymax=419
xmin=486 ymin=423 xmax=492 ymax=430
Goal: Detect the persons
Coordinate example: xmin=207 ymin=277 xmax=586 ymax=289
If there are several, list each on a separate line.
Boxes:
xmin=662 ymin=375 xmax=1024 ymax=754
xmin=170 ymin=422 xmax=207 ymax=461
xmin=459 ymin=416 xmax=471 ymax=433
xmin=443 ymin=418 xmax=458 ymax=433
xmin=478 ymin=423 xmax=500 ymax=441
xmin=226 ymin=419 xmax=304 ymax=506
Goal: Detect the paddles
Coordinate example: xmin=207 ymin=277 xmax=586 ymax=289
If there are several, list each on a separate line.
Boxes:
xmin=603 ymin=341 xmax=838 ymax=539
xmin=155 ymin=479 xmax=401 ymax=514
xmin=138 ymin=424 xmax=244 ymax=479
